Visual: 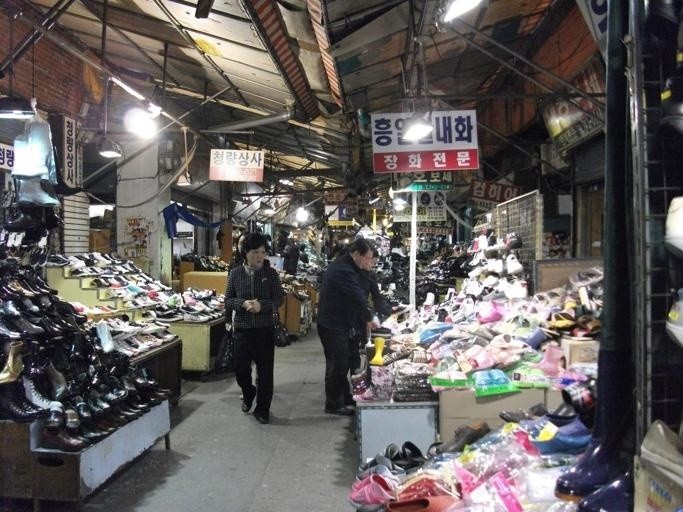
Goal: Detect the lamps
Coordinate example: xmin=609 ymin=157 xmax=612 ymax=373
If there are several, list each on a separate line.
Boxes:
xmin=176 ymin=126 xmax=192 ymax=186
xmin=434 ymin=0 xmax=482 ymax=33
xmin=402 ymin=35 xmax=434 ymax=141
xmin=99 ymin=140 xmax=123 ymax=158
xmin=0 ymin=8 xmax=38 ymax=119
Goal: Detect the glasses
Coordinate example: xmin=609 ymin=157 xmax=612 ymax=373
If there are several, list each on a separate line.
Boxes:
xmin=0 ymin=8 xmax=38 ymax=119
xmin=176 ymin=126 xmax=192 ymax=186
xmin=434 ymin=0 xmax=482 ymax=33
xmin=402 ymin=35 xmax=434 ymax=141
xmin=99 ymin=140 xmax=123 ymax=158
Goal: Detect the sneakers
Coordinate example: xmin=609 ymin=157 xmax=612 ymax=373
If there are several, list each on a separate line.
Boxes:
xmin=194 ymin=228 xmax=310 ymax=298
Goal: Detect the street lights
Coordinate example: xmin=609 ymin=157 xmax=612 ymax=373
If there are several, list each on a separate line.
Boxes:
xmin=271 ymin=313 xmax=291 ymax=347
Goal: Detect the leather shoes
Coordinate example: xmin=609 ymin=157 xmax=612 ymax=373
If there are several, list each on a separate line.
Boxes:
xmin=0 ymin=176 xmax=225 ymax=453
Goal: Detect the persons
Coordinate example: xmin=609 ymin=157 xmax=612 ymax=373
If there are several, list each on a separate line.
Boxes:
xmin=260 ymin=231 xmax=406 ymax=281
xmin=315 ymin=238 xmax=381 ymax=416
xmin=223 ymin=233 xmax=286 ymax=425
xmin=358 ymin=244 xmax=403 ymax=347
xmin=224 ymin=232 xmax=259 ymax=400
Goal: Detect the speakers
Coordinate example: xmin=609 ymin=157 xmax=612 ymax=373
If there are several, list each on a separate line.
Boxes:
xmin=256 ymin=405 xmax=269 ymax=424
xmin=324 ymin=397 xmax=355 ymax=416
xmin=242 ymin=386 xmax=256 ymax=412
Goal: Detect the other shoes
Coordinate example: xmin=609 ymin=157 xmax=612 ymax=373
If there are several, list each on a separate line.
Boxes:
xmin=640 ymin=420 xmax=681 ymax=474
xmin=666 ymin=302 xmax=681 ymax=342
xmin=664 ymin=197 xmax=681 ymax=251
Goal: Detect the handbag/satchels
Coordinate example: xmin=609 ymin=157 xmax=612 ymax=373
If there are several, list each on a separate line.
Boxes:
xmin=271 ymin=313 xmax=291 ymax=347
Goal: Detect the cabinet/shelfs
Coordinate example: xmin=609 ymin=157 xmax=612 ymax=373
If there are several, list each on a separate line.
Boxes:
xmin=354 ymin=246 xmax=600 ymax=467
xmin=1 ymin=258 xmax=170 ymax=507
xmin=40 ymin=259 xmax=225 ymax=406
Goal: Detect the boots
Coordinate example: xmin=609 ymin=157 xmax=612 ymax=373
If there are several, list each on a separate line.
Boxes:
xmin=242 ymin=386 xmax=256 ymax=412
xmin=256 ymin=405 xmax=269 ymax=424
xmin=324 ymin=397 xmax=355 ymax=416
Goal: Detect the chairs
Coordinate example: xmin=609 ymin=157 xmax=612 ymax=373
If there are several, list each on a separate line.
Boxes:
xmin=194 ymin=228 xmax=310 ymax=298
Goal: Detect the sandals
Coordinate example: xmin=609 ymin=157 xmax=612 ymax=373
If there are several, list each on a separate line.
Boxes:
xmin=349 ymin=233 xmax=627 ymax=512
